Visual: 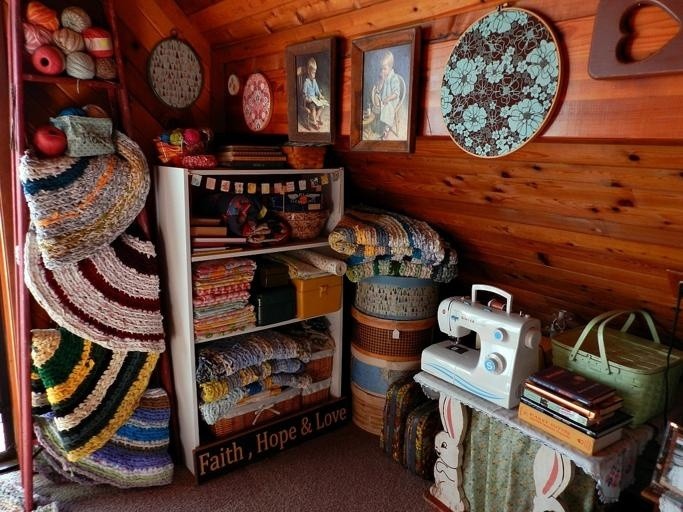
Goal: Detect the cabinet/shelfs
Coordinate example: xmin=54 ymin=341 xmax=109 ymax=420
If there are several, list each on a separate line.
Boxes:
xmin=5 ymin=0 xmax=182 ymax=512
xmin=152 ymin=165 xmax=346 ymax=476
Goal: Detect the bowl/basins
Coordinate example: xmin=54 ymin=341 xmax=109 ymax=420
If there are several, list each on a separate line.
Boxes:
xmin=271 ymin=208 xmax=332 ymax=242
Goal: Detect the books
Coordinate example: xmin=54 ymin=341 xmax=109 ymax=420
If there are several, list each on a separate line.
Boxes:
xmin=220 ymin=144 xmax=288 ymax=168
xmin=515 ymin=368 xmax=634 ymax=456
xmin=191 ymin=216 xmax=247 ymax=256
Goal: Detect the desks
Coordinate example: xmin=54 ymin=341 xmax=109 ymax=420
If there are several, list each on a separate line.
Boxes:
xmin=412 ymin=373 xmax=655 ymax=512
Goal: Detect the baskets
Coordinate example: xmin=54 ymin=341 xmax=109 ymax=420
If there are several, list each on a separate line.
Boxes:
xmin=548 ymin=310 xmax=681 ymax=428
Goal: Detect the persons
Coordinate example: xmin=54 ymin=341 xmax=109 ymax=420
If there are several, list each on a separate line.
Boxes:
xmin=302 ymin=57 xmax=325 ymax=131
xmin=362 ymin=51 xmax=399 ymax=137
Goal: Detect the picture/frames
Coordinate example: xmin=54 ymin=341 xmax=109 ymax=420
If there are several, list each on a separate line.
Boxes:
xmin=649 ymin=419 xmax=683 ymax=503
xmin=286 ymin=26 xmax=421 ymax=154
